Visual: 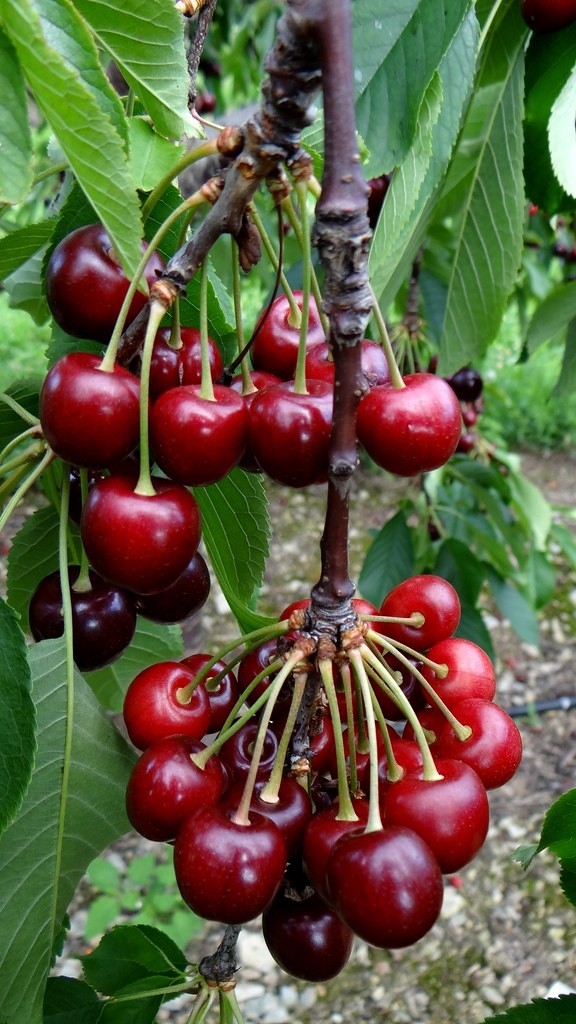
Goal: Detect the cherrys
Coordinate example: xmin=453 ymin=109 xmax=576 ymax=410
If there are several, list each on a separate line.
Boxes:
xmin=28 ymin=129 xmax=522 ymax=981
